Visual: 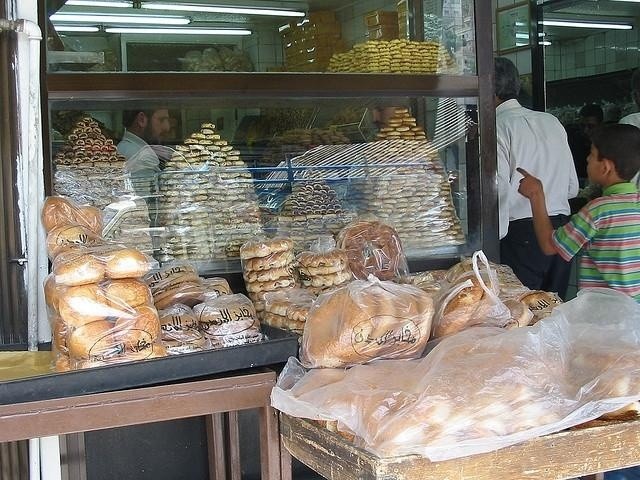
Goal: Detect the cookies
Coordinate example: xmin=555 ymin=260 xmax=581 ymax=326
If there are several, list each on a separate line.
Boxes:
xmin=52 ymin=39 xmax=466 ymax=264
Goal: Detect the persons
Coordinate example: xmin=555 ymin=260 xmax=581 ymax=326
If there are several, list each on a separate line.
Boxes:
xmin=113 ymin=107 xmax=170 ymax=203
xmin=514 ymin=121 xmax=640 ymax=308
xmin=562 ymin=124 xmax=592 ymax=189
xmin=230 ymin=114 xmax=267 ymax=161
xmin=158 ymin=115 xmax=180 ymax=147
xmin=495 ymin=54 xmax=580 ymax=303
xmin=348 ymin=99 xmax=411 ymax=210
xmin=577 ymin=104 xmax=603 ymax=143
xmin=617 ymin=68 xmax=640 ymax=186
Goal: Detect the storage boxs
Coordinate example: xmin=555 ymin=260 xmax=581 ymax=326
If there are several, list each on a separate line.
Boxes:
xmin=278 ymin=10 xmax=347 ymax=73
xmin=364 ymin=1 xmax=408 ymax=38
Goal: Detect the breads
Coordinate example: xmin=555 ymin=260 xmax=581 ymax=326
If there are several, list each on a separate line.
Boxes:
xmin=41 ymin=196 xmax=640 ymax=460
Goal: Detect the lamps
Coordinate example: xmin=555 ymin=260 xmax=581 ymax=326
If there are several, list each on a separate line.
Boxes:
xmin=516 ymin=20 xmax=633 ymax=47
xmin=44 ymin=0 xmax=307 ymax=35
